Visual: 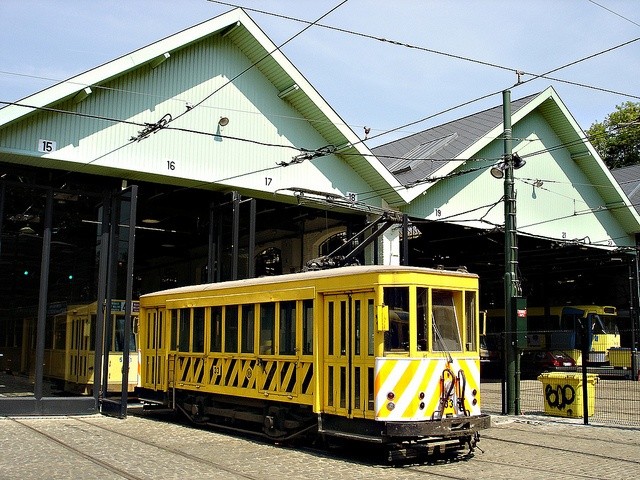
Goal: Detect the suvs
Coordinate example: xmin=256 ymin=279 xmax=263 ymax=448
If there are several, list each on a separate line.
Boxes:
xmin=520 ymin=348 xmax=577 ymax=377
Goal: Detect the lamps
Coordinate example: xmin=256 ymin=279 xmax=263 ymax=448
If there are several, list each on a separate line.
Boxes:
xmin=533 ymin=178 xmax=543 ymax=188
xmin=216 ymin=114 xmax=230 ymax=128
xmin=491 ymin=163 xmax=506 ymax=179
xmin=512 ymin=151 xmax=526 ymax=170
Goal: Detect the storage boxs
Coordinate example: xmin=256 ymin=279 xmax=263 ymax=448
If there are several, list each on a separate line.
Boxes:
xmin=537 ymin=371 xmax=600 ymax=419
xmin=607 ymin=348 xmax=634 ymax=369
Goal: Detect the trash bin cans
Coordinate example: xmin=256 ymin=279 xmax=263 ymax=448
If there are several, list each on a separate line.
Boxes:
xmin=536 ymin=370 xmax=598 ymax=419
xmin=607 ymin=347 xmax=637 ymax=369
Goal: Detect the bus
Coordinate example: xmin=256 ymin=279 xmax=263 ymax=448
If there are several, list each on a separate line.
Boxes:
xmin=136 ymin=265 xmax=490 ymax=466
xmin=478 ymin=304 xmax=620 ymax=366
xmin=1 ymin=296 xmax=140 ymax=396
